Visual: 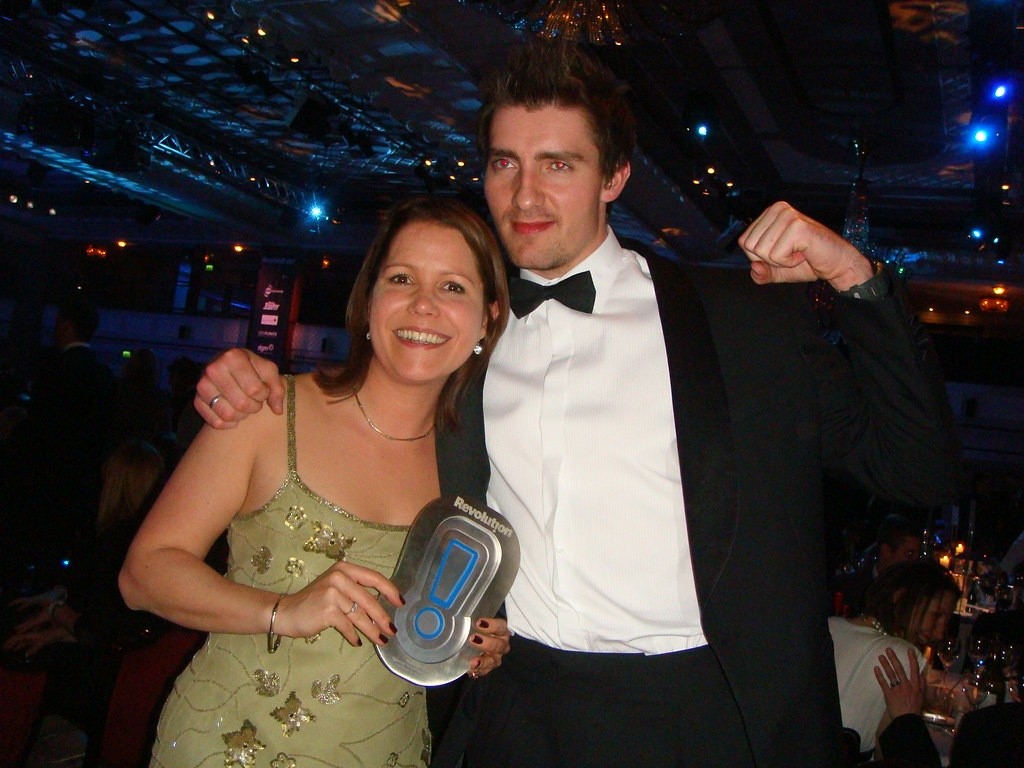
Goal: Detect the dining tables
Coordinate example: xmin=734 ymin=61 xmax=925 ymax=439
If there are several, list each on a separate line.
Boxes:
xmin=917 ymin=671 xmax=1015 ymax=768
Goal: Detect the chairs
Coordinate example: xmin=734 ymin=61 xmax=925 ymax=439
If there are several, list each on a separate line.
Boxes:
xmin=0 ymin=622 xmax=209 ymax=768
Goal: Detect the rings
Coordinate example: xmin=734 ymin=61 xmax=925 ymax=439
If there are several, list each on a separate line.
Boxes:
xmin=344 ymin=602 xmax=358 ymax=615
xmin=890 ymin=681 xmax=899 ymax=686
xmin=468 ymin=670 xmax=479 ymax=677
xmin=209 ymin=394 xmax=224 ymax=408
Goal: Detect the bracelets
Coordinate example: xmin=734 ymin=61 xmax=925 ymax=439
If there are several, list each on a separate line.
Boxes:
xmin=268 ymin=593 xmax=283 ymax=654
xmin=49 ymin=600 xmax=65 ymax=623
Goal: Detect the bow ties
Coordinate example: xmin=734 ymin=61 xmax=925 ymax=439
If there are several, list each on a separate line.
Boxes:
xmin=509 ymin=271 xmax=596 ymax=319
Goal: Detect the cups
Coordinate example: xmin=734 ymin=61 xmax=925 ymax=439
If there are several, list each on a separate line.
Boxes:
xmin=998 ymin=591 xmax=1013 ymax=611
xmin=988 ymin=633 xmax=1017 ymax=669
xmin=964 ymin=635 xmax=990 ymax=668
xmin=839 ymin=544 xmax=880 ymax=594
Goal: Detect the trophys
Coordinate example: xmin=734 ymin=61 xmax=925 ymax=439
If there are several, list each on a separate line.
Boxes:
xmin=374 ymin=495 xmax=521 ymax=688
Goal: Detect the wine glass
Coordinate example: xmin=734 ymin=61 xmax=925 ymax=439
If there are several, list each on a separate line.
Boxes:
xmin=935 ymin=638 xmax=962 ymax=692
xmin=931 ymin=671 xmax=993 ymax=738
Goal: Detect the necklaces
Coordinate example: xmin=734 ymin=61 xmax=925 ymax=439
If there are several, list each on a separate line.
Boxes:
xmin=353 ymin=388 xmax=435 ymax=441
xmin=863 ymin=617 xmax=887 ymax=636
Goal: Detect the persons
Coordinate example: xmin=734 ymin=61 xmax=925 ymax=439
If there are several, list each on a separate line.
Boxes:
xmin=118 ymin=196 xmax=510 ymax=767
xmin=193 ymin=34 xmax=949 ymax=768
xmin=826 ymin=516 xmax=1024 ymax=768
xmin=0 ymin=305 xmax=205 ymax=768
